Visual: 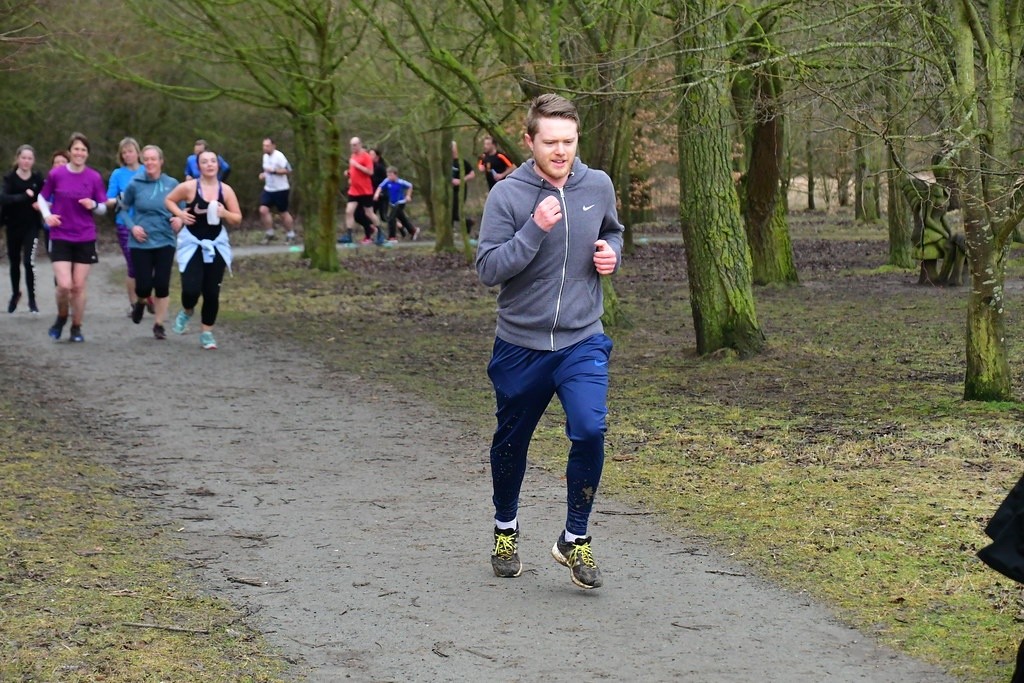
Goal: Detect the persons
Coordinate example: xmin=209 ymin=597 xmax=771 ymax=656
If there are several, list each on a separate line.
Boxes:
xmin=975 ymin=470 xmax=1024 ymax=683
xmin=106 ymin=137 xmax=157 ymax=317
xmin=926 ymin=153 xmax=967 ymax=287
xmin=441 ymin=141 xmax=476 ymax=236
xmin=121 ymin=145 xmax=186 ymax=340
xmin=257 ymin=139 xmax=300 ymax=243
xmin=0 ymin=145 xmax=51 ymax=314
xmin=469 ymin=134 xmax=514 ymax=249
xmin=185 ymin=140 xmax=232 ymax=184
xmin=336 ymin=136 xmax=421 ymax=248
xmin=475 ymin=95 xmax=626 ymax=592
xmin=36 ymin=133 xmax=110 ymax=343
xmin=165 ymin=151 xmax=242 ymax=348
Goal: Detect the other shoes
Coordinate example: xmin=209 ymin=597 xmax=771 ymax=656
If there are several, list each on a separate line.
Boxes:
xmin=27 ymin=300 xmax=39 ymax=313
xmin=260 ymin=235 xmax=276 ymax=244
xmin=387 ymin=237 xmax=397 ymax=243
xmin=284 ymin=232 xmax=296 ymax=246
xmin=126 ymin=302 xmax=134 ymax=317
xmin=467 ymin=218 xmax=476 ymax=234
xmin=361 ymin=237 xmax=374 ymax=244
xmin=400 ymin=224 xmax=408 ymax=239
xmin=338 ymin=234 xmax=352 ymax=242
xmin=8 ymin=291 xmax=22 ymax=314
xmin=147 ymin=295 xmax=157 ymax=315
xmin=411 ymin=227 xmax=421 ymax=241
xmin=376 ymin=231 xmax=386 ymax=245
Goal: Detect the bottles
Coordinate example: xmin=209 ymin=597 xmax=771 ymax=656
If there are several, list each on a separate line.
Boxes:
xmin=207 ymin=201 xmax=220 ymax=224
xmin=491 ymin=169 xmax=498 ymax=179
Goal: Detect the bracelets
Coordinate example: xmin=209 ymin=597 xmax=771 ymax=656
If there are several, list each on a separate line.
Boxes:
xmin=93 ymin=200 xmax=98 ymax=210
xmin=274 ymin=168 xmax=276 ymax=173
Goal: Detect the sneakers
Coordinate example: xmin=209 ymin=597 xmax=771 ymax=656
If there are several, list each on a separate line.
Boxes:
xmin=131 ymin=298 xmax=146 ymax=323
xmin=70 ymin=328 xmax=83 ymax=342
xmin=172 ymin=309 xmax=194 ymax=333
xmin=47 ymin=314 xmax=67 ymax=340
xmin=153 ymin=323 xmax=165 ymax=339
xmin=200 ymin=331 xmax=216 ymax=350
xmin=490 ymin=523 xmax=522 ymax=577
xmin=552 ymin=528 xmax=602 ymax=589
